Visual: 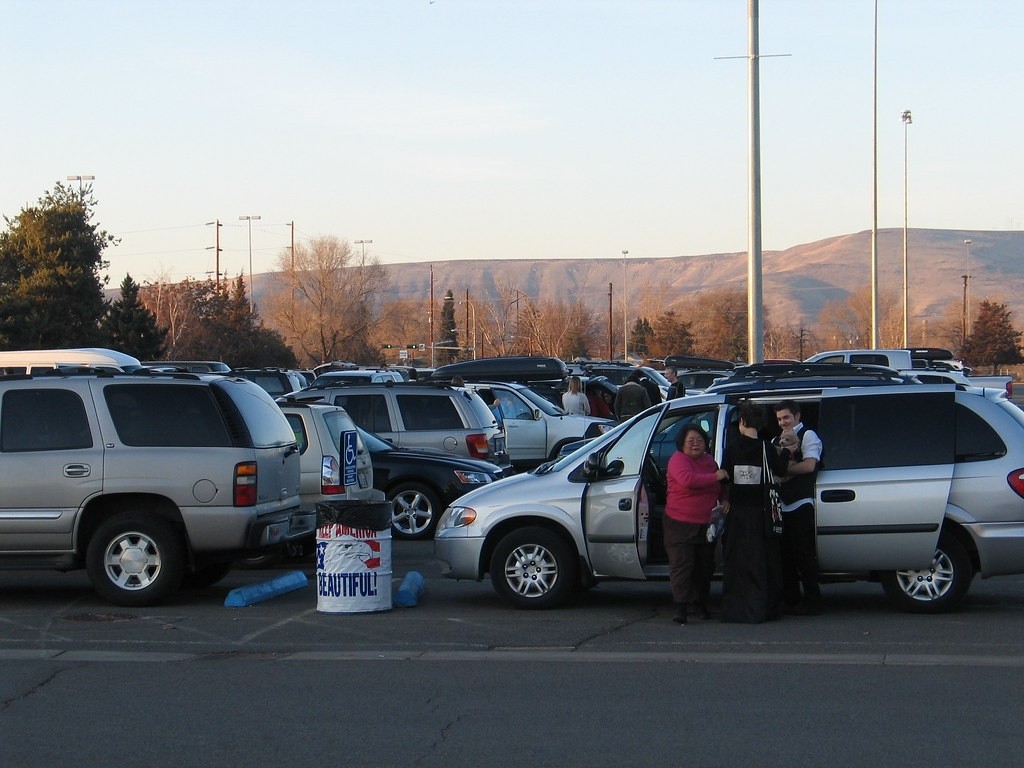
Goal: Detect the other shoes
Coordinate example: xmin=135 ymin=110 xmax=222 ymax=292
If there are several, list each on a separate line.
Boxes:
xmin=790 ymin=593 xmax=802 ymax=606
xmin=794 ymin=598 xmax=823 ymax=615
xmin=695 ymin=604 xmax=711 ymax=620
xmin=673 ymin=609 xmax=687 ymax=623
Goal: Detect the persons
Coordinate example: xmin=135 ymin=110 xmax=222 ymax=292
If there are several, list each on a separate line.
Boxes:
xmin=408 ymin=369 xmax=420 ymax=383
xmin=586 ymin=369 xmax=662 ymax=424
xmin=718 ymin=400 xmax=822 ymax=617
xmin=665 ymin=367 xmax=685 ymax=401
xmin=664 ymin=425 xmax=729 ymax=622
xmin=562 ymin=377 xmax=591 ymax=417
xmin=451 ymin=375 xmax=500 ymax=411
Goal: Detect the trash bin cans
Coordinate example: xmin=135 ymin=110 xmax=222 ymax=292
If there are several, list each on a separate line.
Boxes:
xmin=314 ymin=498 xmax=394 ymax=614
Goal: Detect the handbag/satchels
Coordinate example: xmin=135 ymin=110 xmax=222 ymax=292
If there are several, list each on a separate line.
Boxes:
xmin=762 ymin=440 xmax=784 ymax=538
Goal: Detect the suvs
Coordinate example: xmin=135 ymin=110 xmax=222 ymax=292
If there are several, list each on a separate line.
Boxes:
xmin=429 ymin=372 xmax=1024 ymax=614
xmin=0 ymin=361 xmax=302 ymax=609
xmin=0 ymin=345 xmax=1024 ymax=575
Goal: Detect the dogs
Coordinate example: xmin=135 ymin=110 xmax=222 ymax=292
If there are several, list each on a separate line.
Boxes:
xmin=775 ymin=427 xmax=801 ymax=455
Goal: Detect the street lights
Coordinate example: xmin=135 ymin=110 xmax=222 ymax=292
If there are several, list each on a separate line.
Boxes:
xmin=239 ymin=216 xmax=261 ymax=327
xmin=354 ymin=239 xmax=372 ymax=300
xmin=67 ymin=175 xmax=96 ymax=208
xmin=902 ymin=108 xmax=912 ymax=352
xmin=621 ymin=250 xmax=629 ymax=363
xmin=964 ymin=239 xmax=973 ymax=342
